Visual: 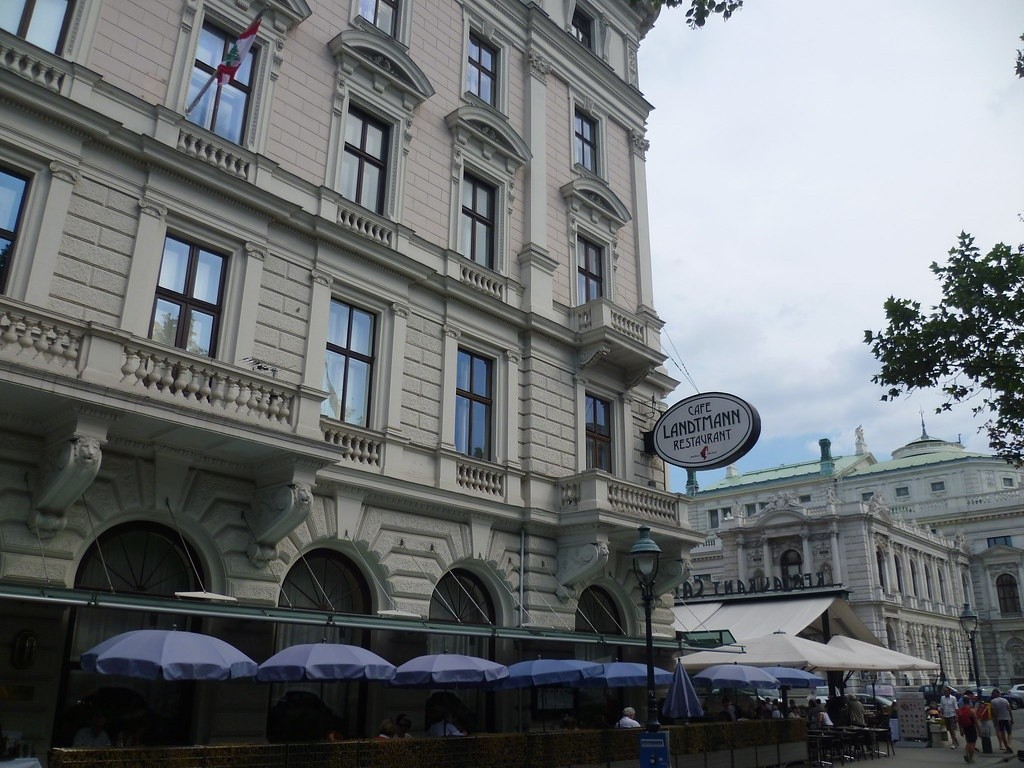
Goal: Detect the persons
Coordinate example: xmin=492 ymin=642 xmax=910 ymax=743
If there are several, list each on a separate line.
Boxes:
xmin=718 ymin=697 xmax=833 ymax=726
xmin=990 ymin=688 xmax=1014 ymax=754
xmin=571 ymin=690 xmax=641 ymax=728
xmin=374 ymin=714 xmax=412 ymax=739
xmin=72 ymin=712 xmax=110 ymax=746
xmin=848 ymin=693 xmax=865 ymax=724
xmin=425 ymin=712 xmax=468 ymax=737
xmin=957 ymin=690 xmax=981 ymax=764
xmin=940 ymin=686 xmax=959 ymax=749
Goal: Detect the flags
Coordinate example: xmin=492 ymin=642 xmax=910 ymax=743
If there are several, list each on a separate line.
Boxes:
xmin=217 ymin=19 xmax=263 ymax=87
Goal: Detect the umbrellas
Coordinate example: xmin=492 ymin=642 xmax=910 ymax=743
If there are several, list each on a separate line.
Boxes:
xmin=385 ymin=649 xmax=509 ymax=737
xmin=81 ymin=624 xmax=258 ymax=681
xmin=257 ymin=638 xmax=396 ymax=700
xmin=677 ymin=629 xmax=940 ymax=672
xmin=760 ymin=664 xmax=826 ymax=686
xmin=662 ymin=658 xmax=704 ymax=719
xmin=693 ymin=661 xmax=780 ymax=707
xmin=552 ymin=658 xmax=673 ymax=689
xmin=482 ymin=654 xmax=604 ymax=732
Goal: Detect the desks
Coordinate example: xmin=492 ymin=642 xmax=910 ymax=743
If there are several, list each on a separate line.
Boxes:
xmin=807 ymin=727 xmax=894 ymax=766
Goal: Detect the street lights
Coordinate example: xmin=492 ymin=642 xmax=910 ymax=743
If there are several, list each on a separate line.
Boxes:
xmin=626 ymin=522 xmax=672 ymax=768
xmin=958 ymin=601 xmax=992 ymax=753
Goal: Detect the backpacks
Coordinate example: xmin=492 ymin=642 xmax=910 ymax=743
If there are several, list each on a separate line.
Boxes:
xmin=959 ymin=709 xmax=973 ymax=727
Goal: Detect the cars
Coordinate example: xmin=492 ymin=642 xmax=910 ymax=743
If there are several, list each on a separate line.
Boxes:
xmin=852 ymin=694 xmax=893 ymax=711
xmin=1007 ymin=684 xmax=1024 ymax=708
xmin=919 ymin=685 xmax=962 ymax=706
xmin=967 ymin=685 xmax=1023 ymax=710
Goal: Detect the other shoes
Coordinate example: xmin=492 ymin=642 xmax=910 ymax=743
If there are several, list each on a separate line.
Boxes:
xmin=950 ymin=744 xmax=956 ymax=749
xmin=963 ymin=754 xmax=970 ymax=763
xmin=1006 ymin=746 xmax=1012 ymax=753
